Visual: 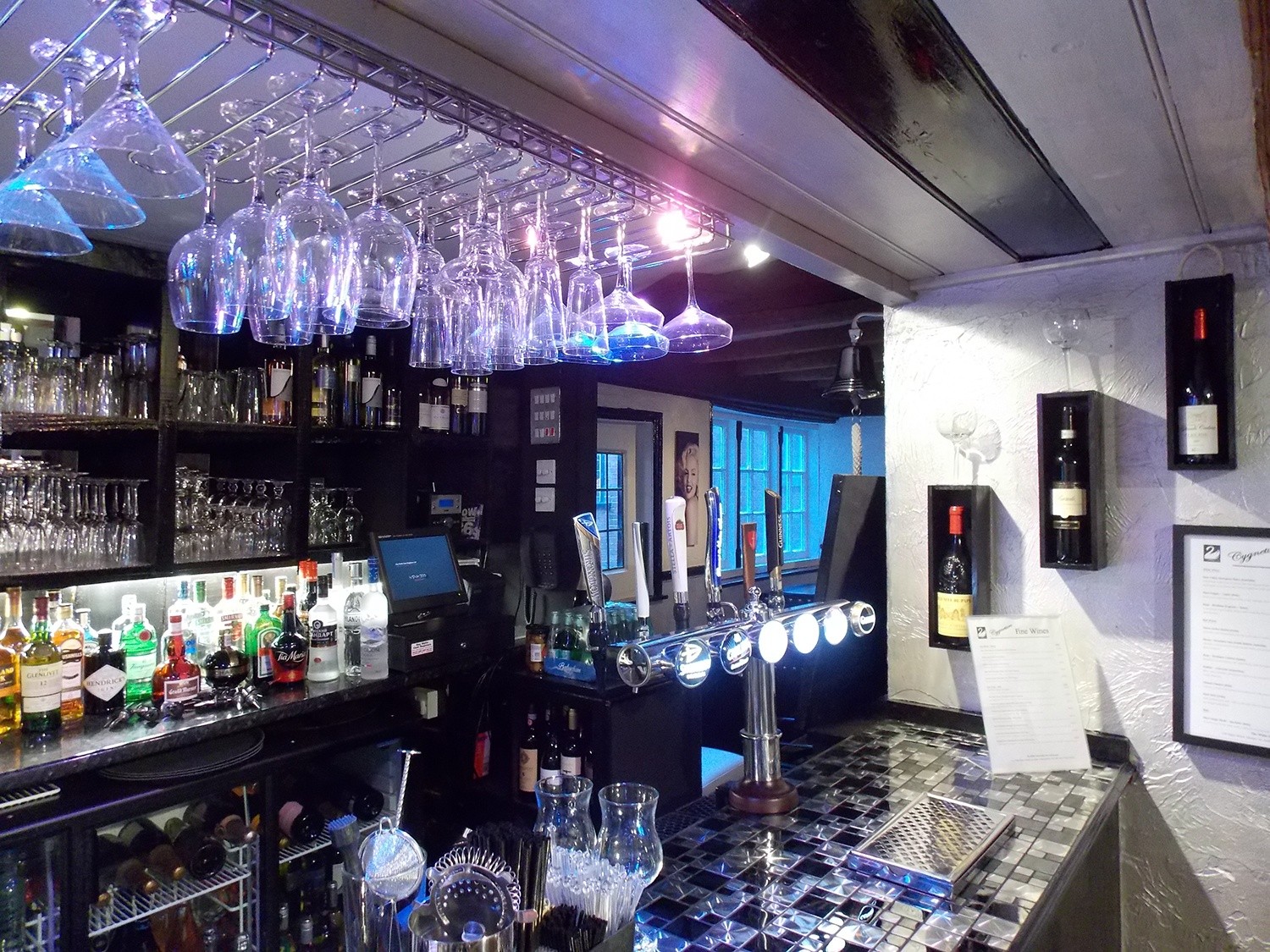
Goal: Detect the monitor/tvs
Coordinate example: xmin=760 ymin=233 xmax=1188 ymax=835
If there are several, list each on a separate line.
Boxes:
xmin=369 ymin=527 xmax=469 ymax=611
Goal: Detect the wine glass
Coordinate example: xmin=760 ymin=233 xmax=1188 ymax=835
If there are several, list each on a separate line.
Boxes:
xmin=937 ymin=405 xmax=977 ymax=484
xmin=1041 ymin=309 xmax=1091 ymax=391
xmin=0 ymin=321 xmax=361 ymax=574
xmin=538 ymin=773 xmax=599 ymax=860
xmin=595 ymin=786 xmax=669 ymax=952
xmin=0 ymin=0 xmax=730 ymax=376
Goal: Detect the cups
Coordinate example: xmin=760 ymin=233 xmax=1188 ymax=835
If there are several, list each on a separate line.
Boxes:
xmin=342 ymin=846 xmax=426 ymax=952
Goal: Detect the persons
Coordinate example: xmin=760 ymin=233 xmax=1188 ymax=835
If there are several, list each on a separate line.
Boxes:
xmin=675 ymin=444 xmax=699 ymax=546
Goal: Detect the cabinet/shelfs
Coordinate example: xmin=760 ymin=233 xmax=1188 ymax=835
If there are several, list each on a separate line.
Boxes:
xmin=5 ymin=238 xmax=604 ymax=951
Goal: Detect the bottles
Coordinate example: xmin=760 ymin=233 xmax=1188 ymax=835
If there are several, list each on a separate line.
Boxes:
xmin=936 ymin=505 xmax=972 ymax=641
xmin=259 ymin=327 xmax=487 ymax=436
xmin=1051 ymin=407 xmax=1090 ymax=564
xmin=1179 ymin=306 xmax=1218 ymax=460
xmin=517 ymin=704 xmax=543 ymax=802
xmin=0 ymin=551 xmax=393 ymax=951
xmin=560 ymin=708 xmax=587 ymax=776
xmin=540 ymin=707 xmax=560 ymax=779
